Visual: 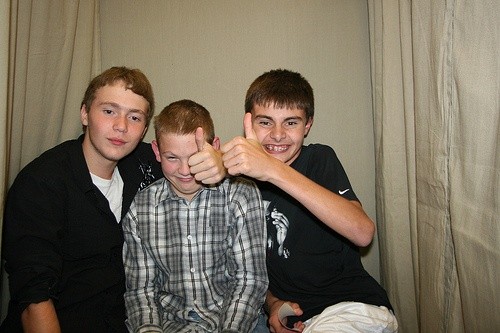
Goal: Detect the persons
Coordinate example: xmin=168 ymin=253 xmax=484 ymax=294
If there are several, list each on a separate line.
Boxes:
xmin=265 ymin=206 xmax=291 ymax=258
xmin=1 ymin=65 xmax=228 ymax=333
xmin=220 ymin=69 xmax=400 ymax=333
xmin=122 ymin=99 xmax=271 ymax=333
